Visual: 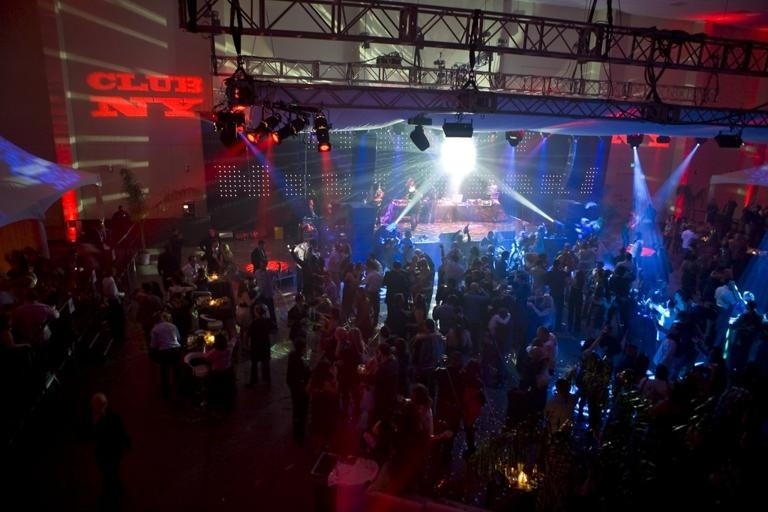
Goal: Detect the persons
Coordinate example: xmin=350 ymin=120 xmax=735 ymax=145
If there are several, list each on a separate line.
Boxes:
xmin=489 ymin=179 xmax=500 ymax=209
xmin=374 ymin=184 xmax=382 ymax=225
xmin=0 ymin=197 xmax=768 ymax=512
xmin=407 ymin=180 xmax=436 ymax=231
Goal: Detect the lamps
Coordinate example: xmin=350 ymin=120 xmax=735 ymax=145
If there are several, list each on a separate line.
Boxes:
xmin=624 ymin=132 xmax=744 ymax=150
xmin=506 ymin=131 xmax=522 ymax=147
xmin=211 ymin=96 xmax=333 ymax=156
xmin=407 ymin=115 xmax=474 ymax=152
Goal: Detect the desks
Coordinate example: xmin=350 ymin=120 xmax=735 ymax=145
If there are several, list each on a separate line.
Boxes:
xmin=269 ymin=268 xmax=297 ymax=289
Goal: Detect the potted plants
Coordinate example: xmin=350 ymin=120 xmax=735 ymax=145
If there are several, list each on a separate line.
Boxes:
xmin=137 ymin=248 xmax=151 ymax=265
